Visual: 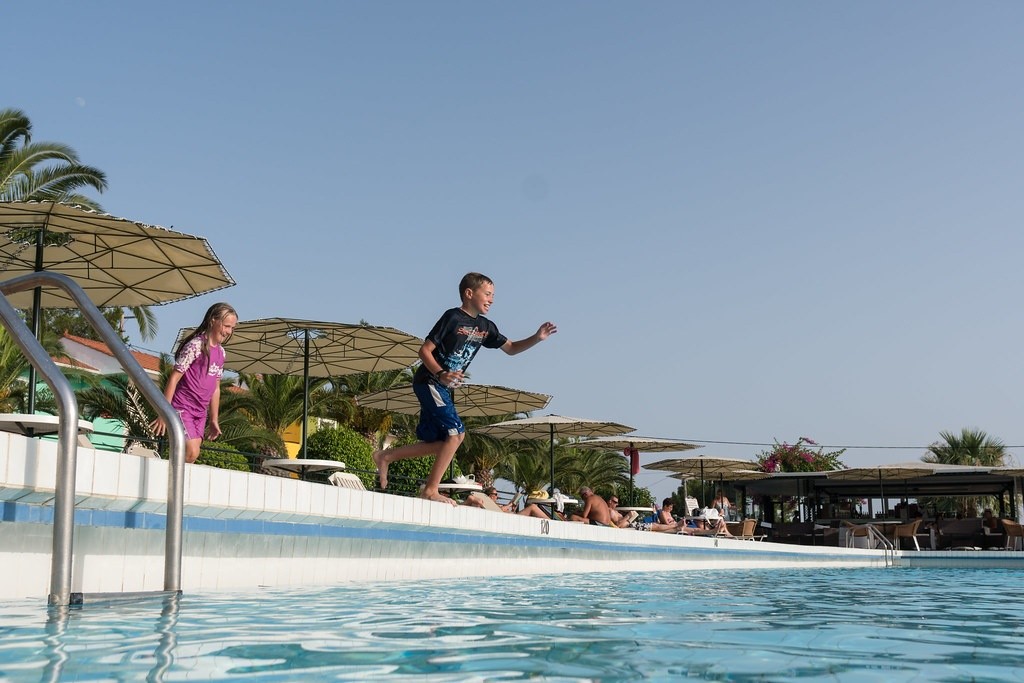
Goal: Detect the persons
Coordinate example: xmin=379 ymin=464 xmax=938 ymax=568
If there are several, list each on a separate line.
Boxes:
xmin=486 ymin=487 xmax=554 ymax=520
xmin=608 ymin=491 xmax=736 ymax=539
xmin=461 ymin=494 xmax=484 ymax=509
xmin=983 ymin=508 xmax=996 ymax=531
xmin=148 ymin=302 xmax=238 ymax=466
xmin=372 ymin=272 xmax=558 ymax=508
xmin=571 ymin=484 xmax=610 ymax=527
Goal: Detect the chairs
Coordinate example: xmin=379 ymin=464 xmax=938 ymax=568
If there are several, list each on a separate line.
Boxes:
xmin=327 ymin=472 xmax=366 ymax=490
xmin=1001 ymin=518 xmax=1024 ymax=550
xmin=730 ymin=519 xmax=757 ymax=541
xmin=842 ymin=518 xmax=875 ymax=550
xmin=895 ymin=520 xmax=922 ymax=551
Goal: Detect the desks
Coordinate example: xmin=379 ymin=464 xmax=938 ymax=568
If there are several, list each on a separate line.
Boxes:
xmin=683 ymin=515 xmax=724 ymax=536
xmin=526 ymin=497 xmax=578 ymax=519
xmin=262 ymin=458 xmax=345 ymax=482
xmin=420 ymin=483 xmax=482 ymax=500
xmin=866 ymin=520 xmax=902 ymax=549
xmin=615 ymin=506 xmax=654 ymax=522
xmin=0 ymin=413 xmax=95 ymax=438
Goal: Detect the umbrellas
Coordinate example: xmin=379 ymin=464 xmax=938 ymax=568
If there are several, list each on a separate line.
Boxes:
xmin=989 ymin=467 xmax=1024 ymax=478
xmin=826 ymin=464 xmax=933 ymax=522
xmin=640 ymin=454 xmax=773 ymax=491
xmin=350 ymin=381 xmax=556 ymax=482
xmin=467 ymin=414 xmax=638 ymax=520
xmin=1 ymin=198 xmax=236 ymax=413
xmin=170 ymin=315 xmax=427 ymax=459
xmin=560 ymin=435 xmax=707 ymax=506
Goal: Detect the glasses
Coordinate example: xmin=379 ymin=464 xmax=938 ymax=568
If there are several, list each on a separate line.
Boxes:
xmin=581 ymin=492 xmax=587 ymax=498
xmin=611 ymin=499 xmax=618 ymax=504
xmin=491 ymin=492 xmax=498 ymax=496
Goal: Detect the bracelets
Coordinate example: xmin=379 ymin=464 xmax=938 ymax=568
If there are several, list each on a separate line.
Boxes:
xmin=434 ymin=369 xmax=447 ymax=381
